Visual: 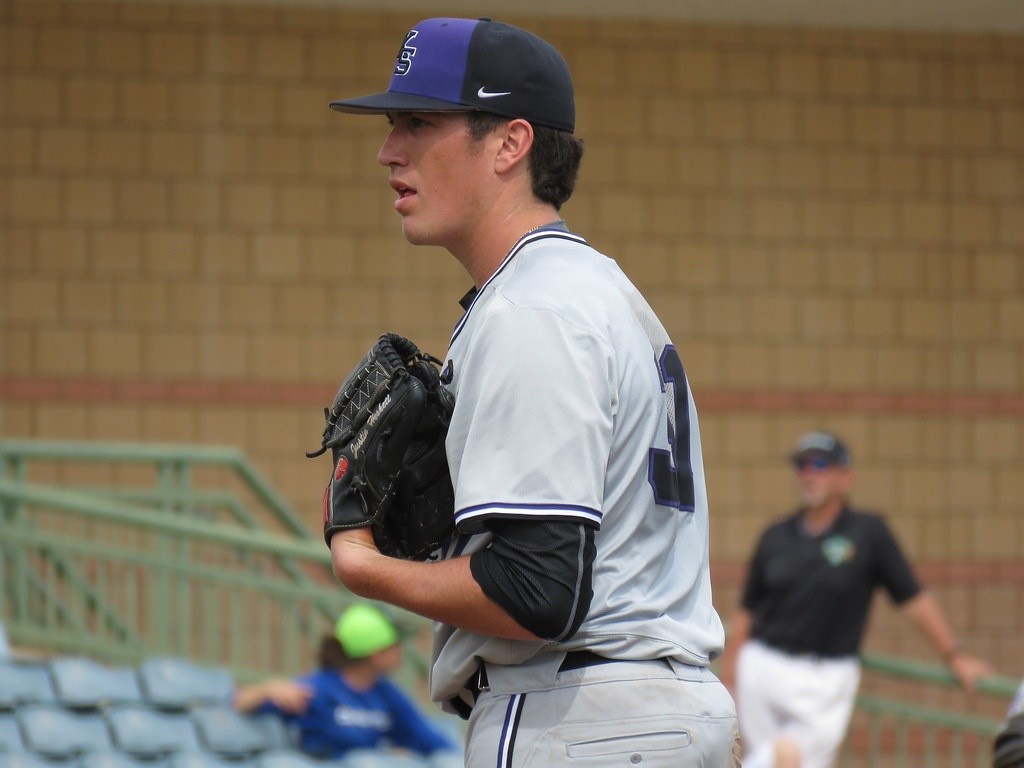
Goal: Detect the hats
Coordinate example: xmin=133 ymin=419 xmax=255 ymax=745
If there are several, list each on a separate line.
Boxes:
xmin=333 ymin=602 xmax=398 ymax=657
xmin=790 ymin=432 xmax=849 ymax=469
xmin=328 ymin=18 xmax=575 ymax=133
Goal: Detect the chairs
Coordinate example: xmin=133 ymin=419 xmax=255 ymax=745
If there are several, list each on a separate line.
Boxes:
xmin=0 ymin=629 xmax=469 ymax=768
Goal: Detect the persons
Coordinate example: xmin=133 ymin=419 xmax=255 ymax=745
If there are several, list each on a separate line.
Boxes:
xmin=237 ymin=601 xmax=459 ymax=768
xmin=719 ymin=427 xmax=995 ymax=767
xmin=324 ymin=18 xmax=736 ymax=768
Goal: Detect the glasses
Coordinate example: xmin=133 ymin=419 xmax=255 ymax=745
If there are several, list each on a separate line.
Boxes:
xmin=794 ymin=455 xmax=835 ymax=471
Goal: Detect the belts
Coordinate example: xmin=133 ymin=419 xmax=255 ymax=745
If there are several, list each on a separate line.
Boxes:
xmin=450 ymin=651 xmax=615 ymax=721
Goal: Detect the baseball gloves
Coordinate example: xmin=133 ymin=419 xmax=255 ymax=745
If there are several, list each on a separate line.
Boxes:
xmin=304 ymin=331 xmax=455 ymax=555
